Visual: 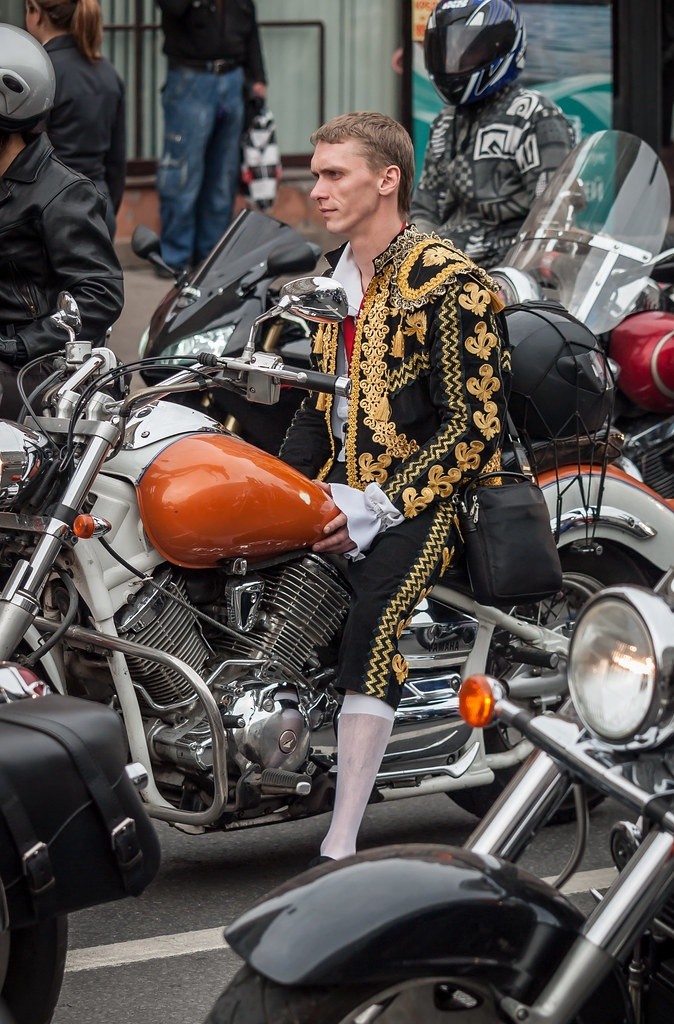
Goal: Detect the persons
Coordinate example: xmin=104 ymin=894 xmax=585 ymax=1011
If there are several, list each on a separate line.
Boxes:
xmin=276 ymin=113 xmax=511 ymax=867
xmin=150 ymin=0 xmax=267 ymax=281
xmin=24 ymin=0 xmax=128 ymax=251
xmin=412 ymin=0 xmax=576 ymax=269
xmin=1 ymin=22 xmax=125 ymax=421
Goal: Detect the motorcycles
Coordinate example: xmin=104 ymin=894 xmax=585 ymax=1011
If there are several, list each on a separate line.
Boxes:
xmin=131 ymin=207 xmax=674 ymax=499
xmin=0 ymin=276 xmax=674 ymax=1024
xmin=203 ymin=562 xmax=674 ymax=1024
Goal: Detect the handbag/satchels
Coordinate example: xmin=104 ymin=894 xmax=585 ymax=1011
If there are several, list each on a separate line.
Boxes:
xmin=240 ymin=103 xmax=284 ymax=211
xmin=454 ymin=414 xmax=564 ymax=607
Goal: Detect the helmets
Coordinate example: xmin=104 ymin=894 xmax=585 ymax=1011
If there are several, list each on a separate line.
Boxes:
xmin=496 ymin=302 xmax=620 ymax=440
xmin=0 ymin=27 xmax=59 ymax=135
xmin=604 ymin=312 xmax=672 ymax=413
xmin=423 ymin=0 xmax=526 ymax=108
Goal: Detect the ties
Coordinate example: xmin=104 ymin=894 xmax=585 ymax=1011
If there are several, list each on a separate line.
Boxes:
xmin=338 ymin=307 xmax=358 ymax=371
